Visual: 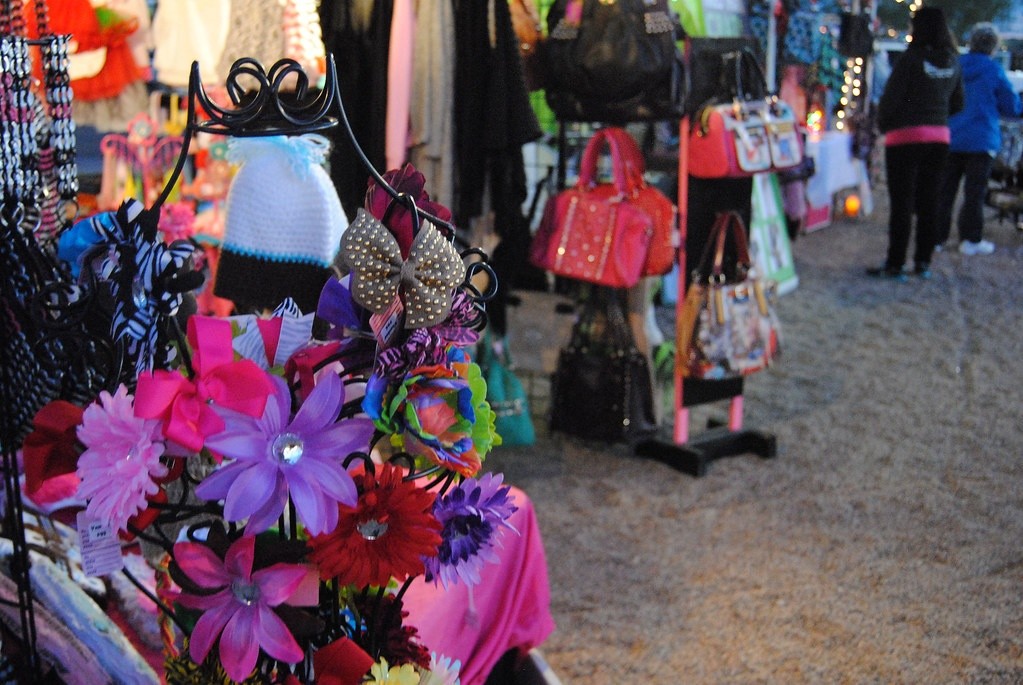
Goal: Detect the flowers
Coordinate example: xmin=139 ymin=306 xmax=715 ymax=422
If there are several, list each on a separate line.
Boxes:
xmin=20 ymin=371 xmax=521 ymax=685
xmin=363 ymin=161 xmax=453 ymax=255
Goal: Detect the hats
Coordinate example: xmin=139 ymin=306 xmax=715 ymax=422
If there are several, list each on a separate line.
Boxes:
xmin=212 ymin=133 xmax=350 ymax=311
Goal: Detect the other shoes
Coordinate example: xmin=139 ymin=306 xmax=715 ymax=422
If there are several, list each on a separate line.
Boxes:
xmin=913 ymin=265 xmax=929 ymax=276
xmin=866 ymin=268 xmax=903 ymax=280
xmin=958 ymin=239 xmax=994 ymax=256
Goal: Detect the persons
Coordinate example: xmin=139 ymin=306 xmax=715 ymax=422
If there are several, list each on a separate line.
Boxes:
xmin=934 ymin=22 xmax=1023 ymax=256
xmin=865 ymin=6 xmax=963 ymax=281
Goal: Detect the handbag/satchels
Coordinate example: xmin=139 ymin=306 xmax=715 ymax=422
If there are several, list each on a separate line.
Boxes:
xmin=0 ymin=226 xmax=118 ymax=454
xmin=478 ymin=324 xmax=537 ymax=444
xmin=676 ymin=213 xmax=782 ymax=378
xmin=688 ymin=47 xmax=802 ymax=177
xmin=549 ymin=290 xmax=663 ymax=441
xmin=543 ymin=127 xmax=680 ymax=288
xmin=534 ymin=0 xmax=685 ymax=122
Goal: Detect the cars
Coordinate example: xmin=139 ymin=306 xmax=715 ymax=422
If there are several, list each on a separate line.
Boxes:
xmin=873 ymin=42 xmax=910 ymax=104
